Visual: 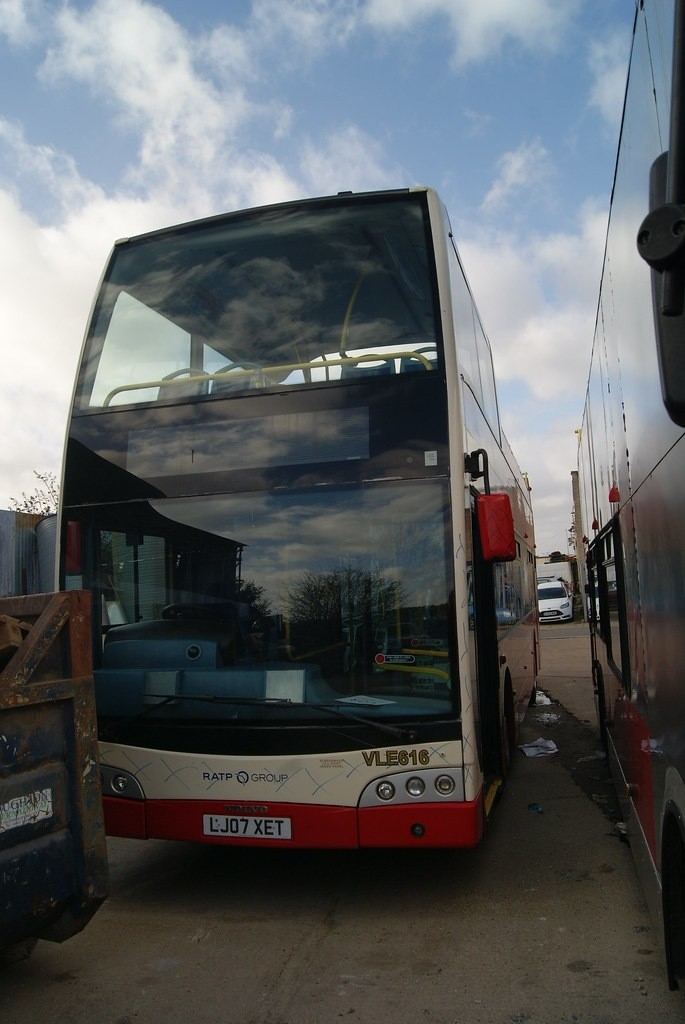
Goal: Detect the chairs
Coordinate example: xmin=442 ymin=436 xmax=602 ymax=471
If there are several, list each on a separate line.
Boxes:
xmin=157 ymin=346 xmax=440 ymax=399
xmin=152 ymin=600 xmax=244 ymax=666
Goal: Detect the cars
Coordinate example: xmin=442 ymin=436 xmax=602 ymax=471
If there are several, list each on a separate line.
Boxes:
xmin=537 ymin=582 xmax=574 ymax=622
xmin=468 ymin=602 xmax=516 ymax=630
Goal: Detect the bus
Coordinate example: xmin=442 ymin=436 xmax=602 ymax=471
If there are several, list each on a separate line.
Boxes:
xmin=582 ymin=0 xmax=685 ymax=991
xmin=56 ymin=183 xmax=541 ymax=848
